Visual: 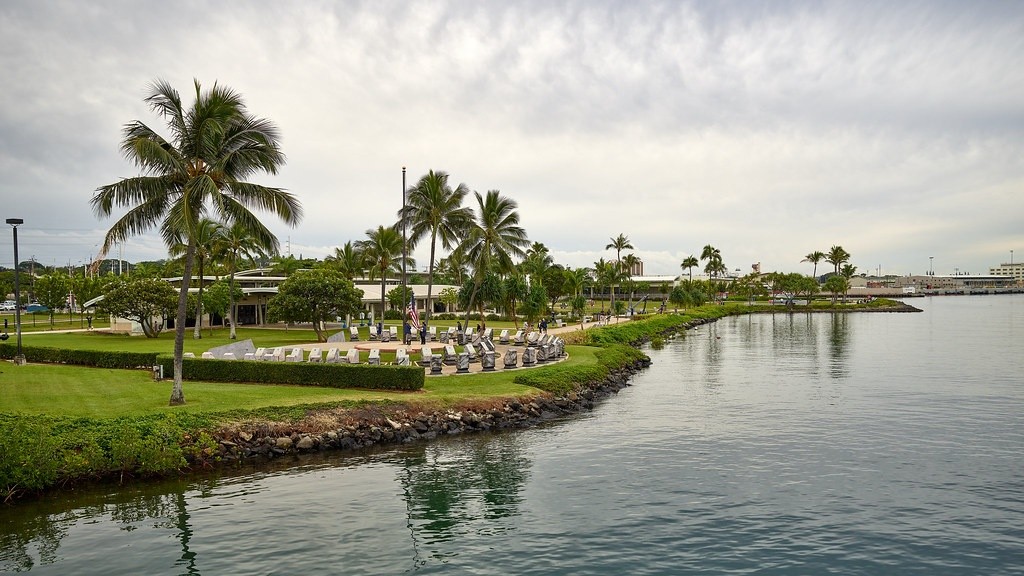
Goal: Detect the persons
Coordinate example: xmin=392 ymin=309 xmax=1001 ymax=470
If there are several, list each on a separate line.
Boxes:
xmin=607 ymin=309 xmax=611 ymax=321
xmin=420 ymin=322 xmax=427 ymax=345
xmin=538 ymin=317 xmax=547 ymax=333
xmin=377 ymin=323 xmax=382 ymax=335
xmin=477 ymin=324 xmax=481 ymax=332
xmin=405 ymin=323 xmax=411 ymax=344
xmin=457 ymin=321 xmax=462 ymax=330
xmin=601 ymin=309 xmax=604 ymax=319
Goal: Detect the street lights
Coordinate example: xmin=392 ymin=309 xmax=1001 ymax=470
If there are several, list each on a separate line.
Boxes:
xmin=6 ymin=218 xmax=23 ymax=358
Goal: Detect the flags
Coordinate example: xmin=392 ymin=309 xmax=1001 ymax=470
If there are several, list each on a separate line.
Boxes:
xmin=409 ymin=297 xmax=419 ymax=330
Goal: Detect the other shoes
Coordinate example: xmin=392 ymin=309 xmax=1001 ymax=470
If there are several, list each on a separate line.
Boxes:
xmin=424 ymin=344 xmax=425 ymax=345
xmin=420 ymin=343 xmax=423 ymax=345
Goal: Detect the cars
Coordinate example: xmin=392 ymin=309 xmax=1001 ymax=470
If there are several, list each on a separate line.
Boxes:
xmin=0 ymin=300 xmax=18 ymax=310
